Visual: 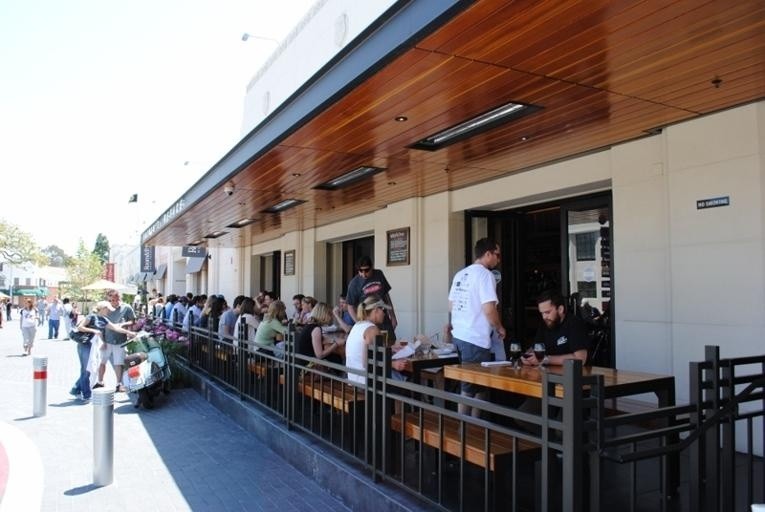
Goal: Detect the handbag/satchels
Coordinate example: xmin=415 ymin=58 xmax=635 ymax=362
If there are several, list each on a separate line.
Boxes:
xmin=67 ymin=328 xmax=94 ymax=344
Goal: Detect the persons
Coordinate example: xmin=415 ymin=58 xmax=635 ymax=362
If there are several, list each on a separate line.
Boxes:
xmin=46 ymin=298 xmax=63 ymax=339
xmin=5 ymin=298 xmax=13 ymax=321
xmin=444 ymin=323 xmax=506 ymax=361
xmin=345 ymin=296 xmax=415 ymax=442
xmin=345 ymin=256 xmax=398 ymax=348
xmin=61 ymin=298 xmax=80 ymax=341
xmin=147 ymin=290 xmax=354 ymax=402
xmin=20 ymin=296 xmax=39 ymax=357
xmin=91 ymin=290 xmax=138 ymax=392
xmin=514 ymin=290 xmax=589 ymax=437
xmin=69 ymin=301 xmax=138 ymax=400
xmin=568 ymin=292 xmax=612 ymax=348
xmin=35 ymin=296 xmax=49 ymax=327
xmin=448 ymin=238 xmax=506 ymax=418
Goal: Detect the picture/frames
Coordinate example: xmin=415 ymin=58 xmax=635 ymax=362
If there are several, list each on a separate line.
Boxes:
xmin=385 ymin=226 xmax=411 ymax=267
xmin=284 ymin=249 xmax=296 ymax=276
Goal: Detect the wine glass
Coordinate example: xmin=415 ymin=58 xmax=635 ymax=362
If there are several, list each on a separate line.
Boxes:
xmin=533 ymin=343 xmax=549 ymax=371
xmin=508 ymin=342 xmax=523 ymax=370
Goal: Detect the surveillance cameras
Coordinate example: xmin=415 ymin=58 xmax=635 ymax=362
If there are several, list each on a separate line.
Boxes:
xmin=224 ymin=186 xmax=234 ymax=195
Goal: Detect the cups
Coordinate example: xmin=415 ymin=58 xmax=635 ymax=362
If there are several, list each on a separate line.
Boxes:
xmin=422 ymin=343 xmax=430 ymax=355
xmin=399 ymin=339 xmax=408 ymax=349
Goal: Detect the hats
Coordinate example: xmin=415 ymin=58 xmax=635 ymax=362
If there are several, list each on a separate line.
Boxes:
xmin=362 ymin=293 xmax=393 ymax=312
xmin=95 ymin=300 xmax=115 ymax=313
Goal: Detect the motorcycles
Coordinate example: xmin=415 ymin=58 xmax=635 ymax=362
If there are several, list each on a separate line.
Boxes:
xmin=119 ymin=331 xmax=173 ymax=409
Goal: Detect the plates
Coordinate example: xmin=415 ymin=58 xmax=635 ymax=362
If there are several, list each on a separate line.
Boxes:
xmin=431 ymin=350 xmax=452 ymax=355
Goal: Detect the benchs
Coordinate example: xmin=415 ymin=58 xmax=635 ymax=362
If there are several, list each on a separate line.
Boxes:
xmin=237 ymin=356 xmax=283 ymax=398
xmin=602 ymin=396 xmax=672 ymax=437
xmin=392 ymin=410 xmax=544 ymax=510
xmin=282 ymin=373 xmax=366 ymax=433
xmin=421 ymin=368 xmax=458 ymax=404
xmin=203 ymin=342 xmax=234 ymax=378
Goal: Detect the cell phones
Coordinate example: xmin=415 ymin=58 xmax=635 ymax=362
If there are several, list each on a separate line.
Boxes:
xmin=522 ymin=353 xmax=532 ymax=358
